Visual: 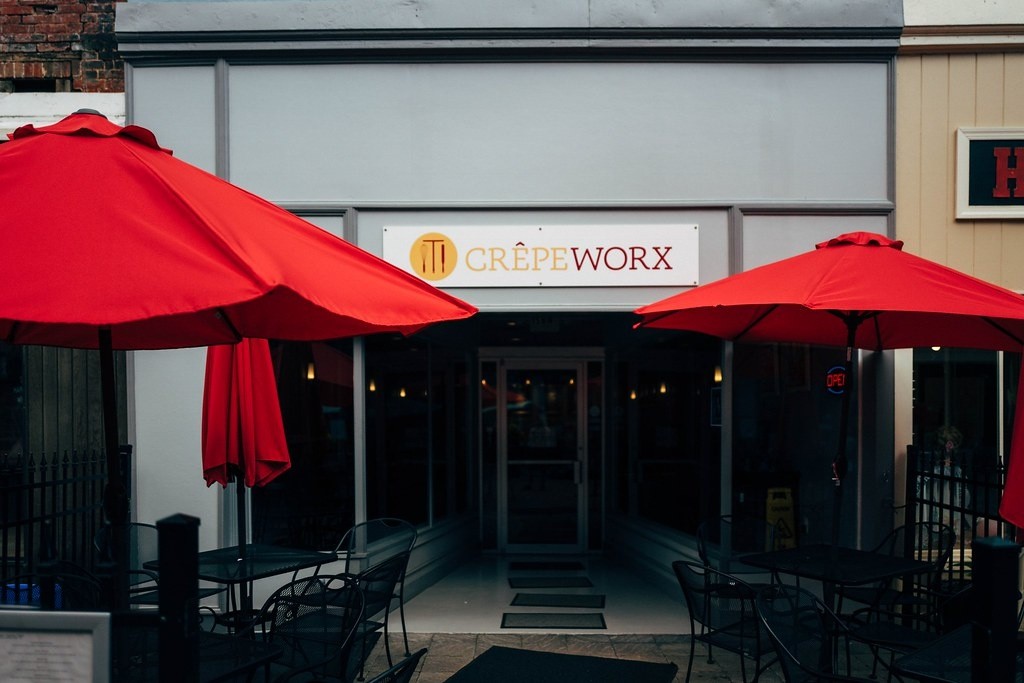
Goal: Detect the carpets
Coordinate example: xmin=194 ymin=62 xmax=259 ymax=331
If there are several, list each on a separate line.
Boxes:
xmin=507 ymin=576 xmax=594 ymax=589
xmin=509 ymin=593 xmax=606 ymax=609
xmin=500 ymin=612 xmax=607 ymax=629
xmin=442 ymin=645 xmax=678 ymax=683
xmin=507 ymin=561 xmax=586 ymax=572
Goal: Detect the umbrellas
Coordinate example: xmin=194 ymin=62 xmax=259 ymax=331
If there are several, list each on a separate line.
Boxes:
xmin=630 ymin=231 xmax=1024 ymax=551
xmin=201 ymin=337 xmax=292 ymax=558
xmin=0 ymin=111 xmax=476 ymax=582
xmin=998 ymin=353 xmax=1024 ymax=530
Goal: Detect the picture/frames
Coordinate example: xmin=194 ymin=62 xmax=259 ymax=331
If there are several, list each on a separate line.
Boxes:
xmin=708 ymin=343 xmax=811 ymax=427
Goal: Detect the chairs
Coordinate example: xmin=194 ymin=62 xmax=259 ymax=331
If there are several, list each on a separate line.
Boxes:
xmin=91 ymin=518 xmax=429 ymax=683
xmin=671 ymin=512 xmax=1024 ymax=683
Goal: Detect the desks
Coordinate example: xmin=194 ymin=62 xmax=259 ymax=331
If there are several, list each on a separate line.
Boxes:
xmin=143 ymin=544 xmax=337 ymax=683
xmin=737 ymin=543 xmax=933 ymax=683
xmin=112 ymin=619 xmax=284 ymax=683
xmin=889 ymin=623 xmax=1024 ymax=683
